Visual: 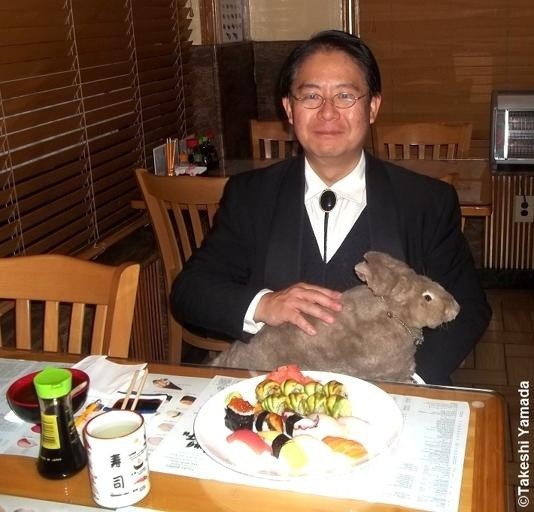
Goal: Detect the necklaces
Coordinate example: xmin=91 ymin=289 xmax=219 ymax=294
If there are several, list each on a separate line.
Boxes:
xmin=376 ymin=297 xmax=425 ymax=345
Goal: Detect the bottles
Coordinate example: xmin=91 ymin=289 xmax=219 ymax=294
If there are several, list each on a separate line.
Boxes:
xmin=33 ymin=365 xmax=87 ymax=480
xmin=180 ymin=133 xmax=221 ymax=171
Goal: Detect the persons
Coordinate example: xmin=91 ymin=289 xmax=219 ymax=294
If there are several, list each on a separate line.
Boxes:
xmin=169 ymin=30 xmax=493 ymax=386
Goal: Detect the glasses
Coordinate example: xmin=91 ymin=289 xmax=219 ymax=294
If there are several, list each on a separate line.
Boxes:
xmin=289 ymin=91 xmax=371 ymax=109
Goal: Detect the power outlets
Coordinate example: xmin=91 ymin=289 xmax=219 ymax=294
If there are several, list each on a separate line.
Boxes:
xmin=515 ymin=195 xmax=534 ymax=223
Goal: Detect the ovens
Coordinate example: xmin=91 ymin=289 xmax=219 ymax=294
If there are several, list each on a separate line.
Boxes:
xmin=492 ymin=92 xmax=534 ymax=172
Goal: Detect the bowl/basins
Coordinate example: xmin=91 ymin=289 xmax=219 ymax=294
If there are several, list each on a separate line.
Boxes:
xmin=6 ymin=367 xmax=89 ymax=424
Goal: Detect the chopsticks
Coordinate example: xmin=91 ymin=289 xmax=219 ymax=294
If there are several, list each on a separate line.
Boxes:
xmin=119 ymin=368 xmax=150 ymax=410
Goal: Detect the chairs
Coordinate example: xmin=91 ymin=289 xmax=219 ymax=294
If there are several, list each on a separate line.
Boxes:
xmin=136 ymin=118 xmax=473 ymax=359
xmin=2 ymin=255 xmax=141 ymax=357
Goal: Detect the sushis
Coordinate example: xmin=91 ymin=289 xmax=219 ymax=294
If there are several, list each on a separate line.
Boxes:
xmin=224 ymin=377 xmax=369 ymax=477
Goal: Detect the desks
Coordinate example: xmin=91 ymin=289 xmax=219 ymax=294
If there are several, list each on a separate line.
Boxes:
xmin=128 ymin=156 xmax=493 ymax=247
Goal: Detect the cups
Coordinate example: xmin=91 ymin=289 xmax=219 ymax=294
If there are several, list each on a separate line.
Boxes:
xmin=84 ymin=409 xmax=150 ymax=509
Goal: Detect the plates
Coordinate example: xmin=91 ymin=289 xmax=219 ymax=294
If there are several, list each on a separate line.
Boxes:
xmin=106 ymin=393 xmax=168 ymax=417
xmin=193 ymin=370 xmax=403 ymax=483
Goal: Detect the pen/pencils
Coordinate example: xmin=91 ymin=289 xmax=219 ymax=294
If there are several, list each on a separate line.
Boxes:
xmin=74 ymin=398 xmax=101 ymax=426
xmin=166 ymin=137 xmax=177 ymax=176
